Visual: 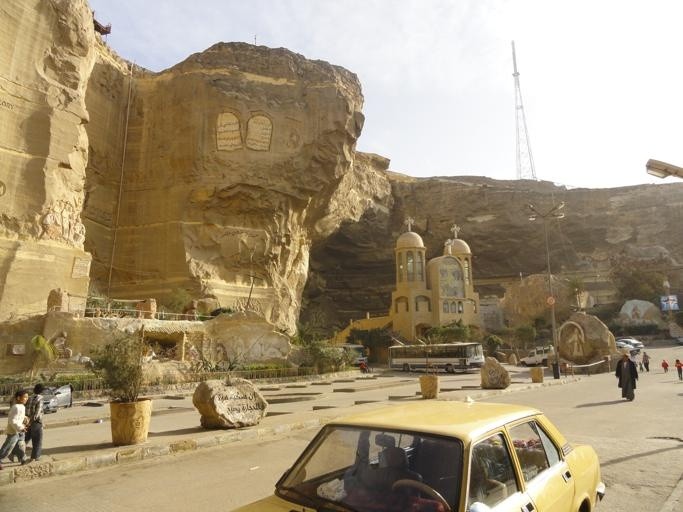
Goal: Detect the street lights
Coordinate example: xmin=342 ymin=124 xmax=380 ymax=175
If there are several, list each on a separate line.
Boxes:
xmin=646 ymin=159 xmax=683 ymax=179
xmin=527 ymin=201 xmax=564 ymax=376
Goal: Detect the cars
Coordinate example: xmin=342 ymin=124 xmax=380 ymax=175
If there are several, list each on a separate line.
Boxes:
xmin=10 ymin=384 xmax=72 ymax=417
xmin=228 ymin=399 xmax=606 ymax=512
xmin=615 ymin=338 xmax=644 ymax=349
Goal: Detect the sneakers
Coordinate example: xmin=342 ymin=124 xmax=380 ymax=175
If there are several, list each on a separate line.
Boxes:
xmin=0 ymin=454 xmax=41 ymax=471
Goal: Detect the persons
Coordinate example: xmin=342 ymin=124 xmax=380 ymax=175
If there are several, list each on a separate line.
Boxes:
xmin=615 ymin=353 xmax=639 ymax=401
xmin=642 ymin=352 xmax=651 ymax=372
xmin=639 ymin=361 xmax=644 ymax=372
xmin=661 ymin=359 xmax=669 ymax=373
xmin=674 ymin=359 xmax=683 ymax=380
xmin=365 ymin=362 xmax=371 ymax=372
xmin=8 ymin=383 xmax=46 ymax=463
xmin=360 ymin=362 xmax=368 ymax=373
xmin=0 ymin=389 xmax=30 ymax=469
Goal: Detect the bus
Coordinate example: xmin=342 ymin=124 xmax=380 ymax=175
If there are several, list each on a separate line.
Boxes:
xmin=388 ymin=343 xmax=485 ymax=373
xmin=334 ymin=343 xmax=368 ymax=367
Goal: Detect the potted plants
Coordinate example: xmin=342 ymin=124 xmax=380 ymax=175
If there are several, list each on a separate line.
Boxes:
xmin=82 ymin=328 xmax=153 ymax=447
xmin=405 ymin=333 xmax=458 ymax=399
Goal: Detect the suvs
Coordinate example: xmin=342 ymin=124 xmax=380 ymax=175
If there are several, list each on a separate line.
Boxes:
xmin=520 ymin=345 xmax=555 ymax=367
